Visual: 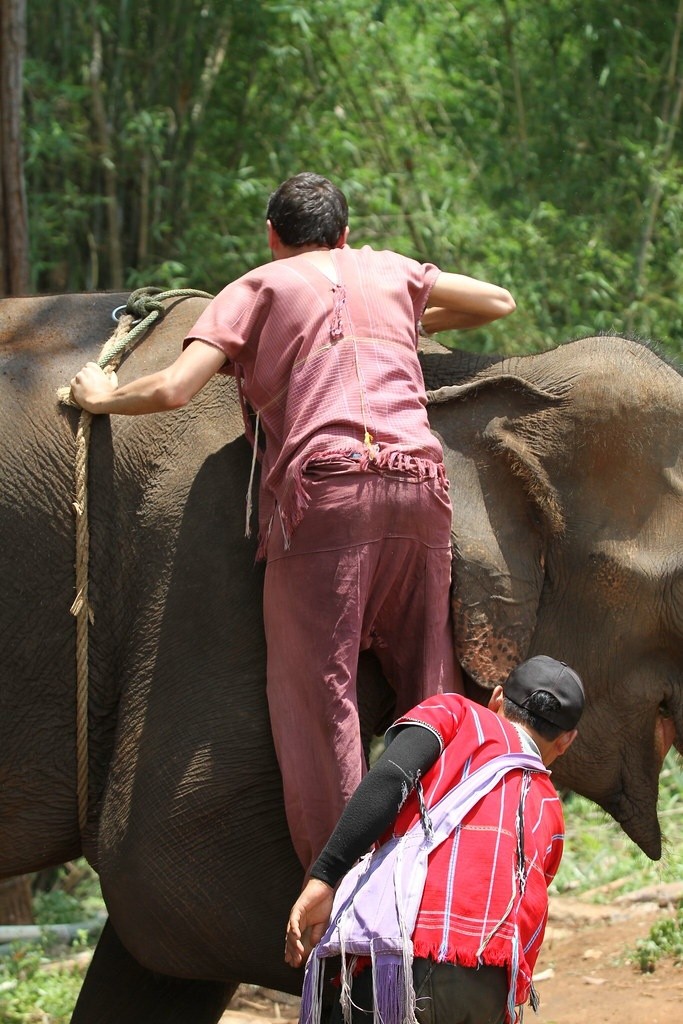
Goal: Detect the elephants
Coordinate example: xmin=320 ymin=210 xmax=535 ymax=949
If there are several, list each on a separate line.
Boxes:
xmin=0 ymin=293 xmax=683 ymax=1023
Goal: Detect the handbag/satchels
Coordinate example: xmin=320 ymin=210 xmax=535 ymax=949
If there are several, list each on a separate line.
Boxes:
xmin=298 ymin=753 xmax=552 ymax=1024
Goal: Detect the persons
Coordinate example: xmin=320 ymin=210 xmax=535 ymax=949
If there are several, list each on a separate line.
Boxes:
xmin=66 ymin=170 xmax=516 ymax=887
xmin=283 ymin=656 xmax=584 ymax=1022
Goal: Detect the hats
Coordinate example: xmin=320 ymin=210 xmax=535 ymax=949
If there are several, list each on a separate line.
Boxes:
xmin=502 ymin=653 xmax=585 ymax=732
